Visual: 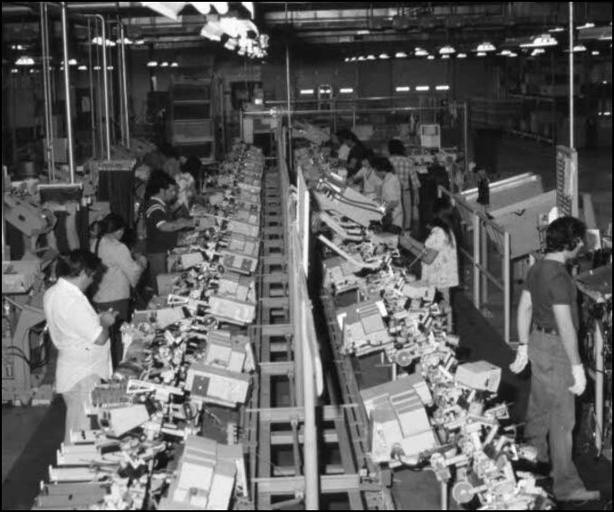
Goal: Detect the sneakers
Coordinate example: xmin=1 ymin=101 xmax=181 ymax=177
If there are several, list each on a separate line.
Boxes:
xmin=552 ymin=484 xmax=602 ymax=501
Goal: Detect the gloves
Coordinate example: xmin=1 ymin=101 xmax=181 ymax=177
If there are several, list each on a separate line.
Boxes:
xmin=516 ymin=343 xmax=531 ymax=351
xmin=569 ymin=363 xmax=587 ymax=399
xmin=509 ymin=347 xmax=530 ymax=376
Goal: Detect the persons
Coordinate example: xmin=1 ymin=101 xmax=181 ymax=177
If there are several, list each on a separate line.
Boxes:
xmin=136 ymin=175 xmax=196 ymax=288
xmin=169 ymin=157 xmax=200 ymax=213
xmin=370 ymin=155 xmax=402 ymax=233
xmin=345 ymin=146 xmax=379 ymax=205
xmin=285 ymin=113 xmax=329 ymax=146
xmin=86 ymin=213 xmax=147 ymax=367
xmin=386 ymin=139 xmax=420 ymax=232
xmin=508 ymin=218 xmax=601 ymax=501
xmin=338 ymin=130 xmax=364 ymax=170
xmin=43 ymin=251 xmax=118 ymax=446
xmin=336 ymin=124 xmax=352 ymax=166
xmin=394 ymin=206 xmax=457 ymax=331
